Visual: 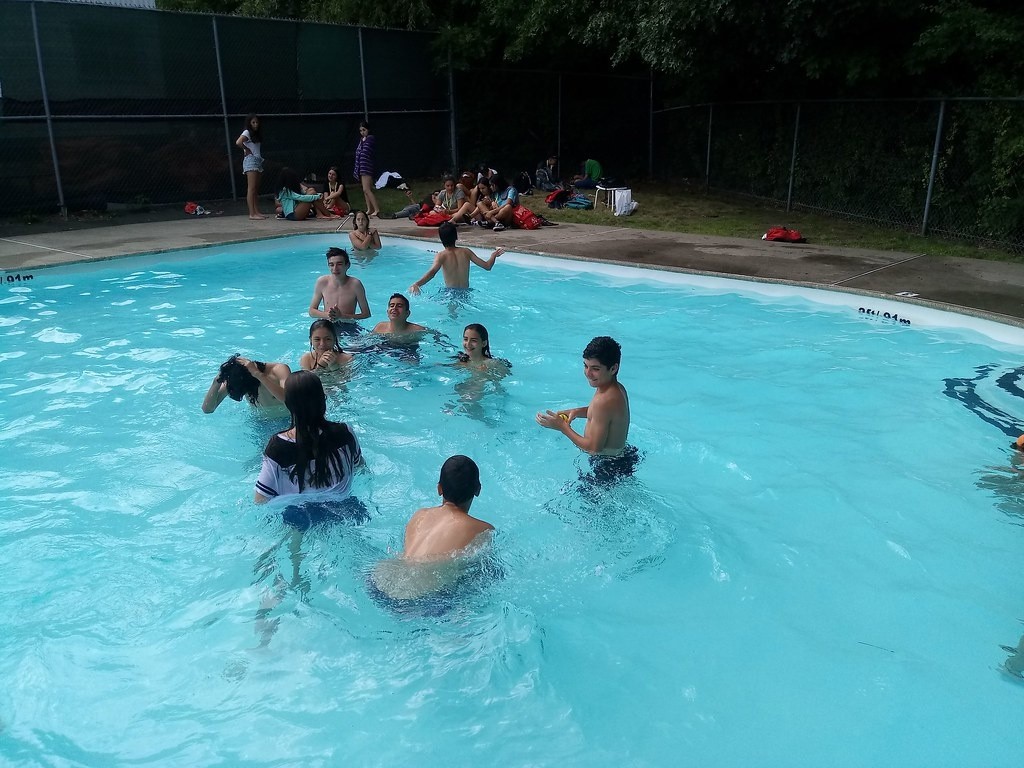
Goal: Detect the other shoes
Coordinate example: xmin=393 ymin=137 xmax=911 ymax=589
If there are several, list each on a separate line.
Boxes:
xmin=216 ymin=209 xmax=225 ymax=215
xmin=462 ymin=214 xmax=473 ymax=226
xmin=493 ymin=222 xmax=506 ymax=231
xmin=476 ymin=220 xmax=489 ymax=227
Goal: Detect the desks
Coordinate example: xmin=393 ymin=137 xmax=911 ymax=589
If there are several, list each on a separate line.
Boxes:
xmin=594 ymin=185 xmax=627 ymax=212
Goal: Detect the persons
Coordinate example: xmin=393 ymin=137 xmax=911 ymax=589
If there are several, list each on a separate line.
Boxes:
xmin=235 ymin=115 xmax=270 ymax=220
xmin=276 ymin=166 xmax=352 ymax=219
xmin=199 ymin=353 xmax=292 ymax=415
xmin=300 ymin=319 xmax=352 ymax=369
xmin=349 ymin=210 xmax=382 ymax=250
xmin=308 ymin=246 xmax=372 ymax=318
xmin=352 ymin=122 xmax=398 ymax=216
xmin=573 ymin=155 xmax=601 ymax=189
xmin=405 ymin=455 xmax=494 ymax=556
xmin=374 ymin=293 xmax=424 ymax=331
xmin=254 ymin=370 xmax=363 ymax=503
xmin=462 ymin=324 xmax=492 ymax=361
xmin=396 ymin=163 xmax=519 ymax=231
xmin=535 ymin=336 xmax=630 ymax=453
xmin=535 ymin=155 xmax=557 ymax=175
xmin=409 ymin=222 xmax=506 ymax=294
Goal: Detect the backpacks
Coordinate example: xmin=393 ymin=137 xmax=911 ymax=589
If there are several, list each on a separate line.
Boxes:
xmin=545 ymin=190 xmax=569 ymax=203
xmin=598 ymin=176 xmax=620 ymax=188
xmin=564 ymin=199 xmax=593 ymax=210
xmin=512 ymin=204 xmax=542 ymax=228
